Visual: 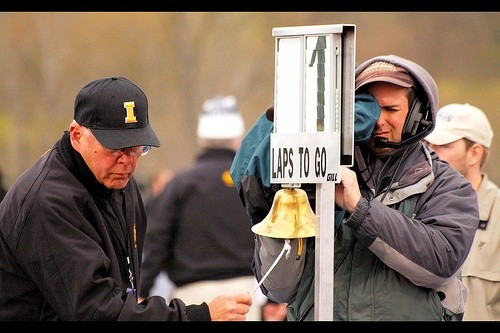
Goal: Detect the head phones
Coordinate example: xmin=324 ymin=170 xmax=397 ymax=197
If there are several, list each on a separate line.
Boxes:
xmin=401 ymin=83 xmax=433 ymax=143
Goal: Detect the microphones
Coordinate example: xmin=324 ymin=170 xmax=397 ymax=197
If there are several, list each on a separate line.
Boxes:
xmin=374 ymin=121 xmax=433 ymax=149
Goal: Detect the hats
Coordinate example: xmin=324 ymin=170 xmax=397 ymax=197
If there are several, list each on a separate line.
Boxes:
xmin=73 ymin=76 xmax=161 ymax=149
xmin=424 ymin=102 xmax=493 ymax=148
xmin=355 ymin=61 xmax=416 ymax=91
xmin=198 ymin=95 xmax=244 ymax=140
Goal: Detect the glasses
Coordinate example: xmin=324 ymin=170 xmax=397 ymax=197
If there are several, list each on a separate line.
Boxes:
xmin=103 ymin=145 xmax=151 ymax=156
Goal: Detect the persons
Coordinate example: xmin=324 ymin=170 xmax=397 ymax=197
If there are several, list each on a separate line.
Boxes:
xmin=230 ymin=55 xmax=478 ymax=321
xmin=0 ymin=76 xmax=253 ymax=321
xmin=418 ymin=103 xmax=500 ymax=321
xmin=140 ymin=95 xmax=269 ymax=321
